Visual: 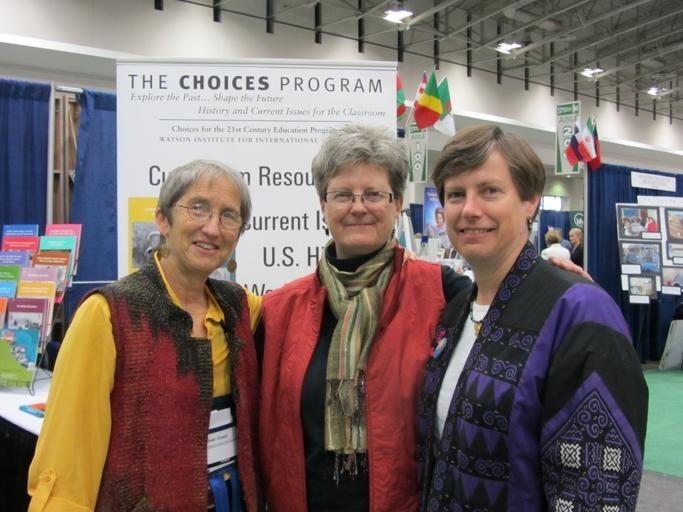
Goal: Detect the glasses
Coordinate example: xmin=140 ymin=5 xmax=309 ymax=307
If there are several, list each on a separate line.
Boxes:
xmin=323 ymin=190 xmax=396 ymax=205
xmin=170 ymin=202 xmax=247 ymax=231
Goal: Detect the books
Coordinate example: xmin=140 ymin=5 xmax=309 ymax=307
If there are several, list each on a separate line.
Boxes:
xmin=0 ymin=224 xmax=82 ymax=372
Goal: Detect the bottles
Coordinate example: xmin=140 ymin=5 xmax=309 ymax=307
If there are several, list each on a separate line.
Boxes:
xmin=418 ymin=236 xmax=428 ymax=261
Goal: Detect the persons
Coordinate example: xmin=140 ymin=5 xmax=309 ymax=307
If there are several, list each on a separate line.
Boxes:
xmin=570 ymin=228 xmax=584 ymax=267
xmin=620 ymin=215 xmax=657 ymax=239
xmin=256 ymin=122 xmax=596 ymax=512
xmin=418 ymin=124 xmax=649 ymax=512
xmin=555 ymin=228 xmax=571 ymax=249
xmin=626 ymin=248 xmax=654 ymax=264
xmin=26 ymin=159 xmax=263 ymax=511
xmin=427 ymin=208 xmax=446 ymax=236
xmin=540 ymin=231 xmax=571 ymax=262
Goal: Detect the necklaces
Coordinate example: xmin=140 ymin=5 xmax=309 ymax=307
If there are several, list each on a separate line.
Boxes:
xmin=468 ymin=299 xmax=489 ymax=336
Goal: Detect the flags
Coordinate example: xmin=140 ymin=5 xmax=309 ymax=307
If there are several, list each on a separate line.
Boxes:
xmin=397 ymin=69 xmax=456 ymax=136
xmin=565 ymin=115 xmax=601 ymax=170
